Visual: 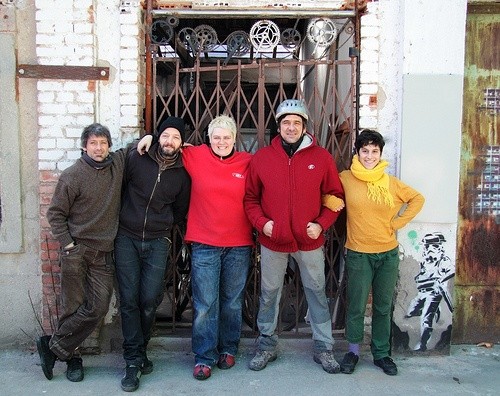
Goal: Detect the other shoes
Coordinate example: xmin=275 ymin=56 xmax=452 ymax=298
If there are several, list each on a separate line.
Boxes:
xmin=66 ymin=358 xmax=84 ymax=382
xmin=36 ymin=335 xmax=56 ymax=380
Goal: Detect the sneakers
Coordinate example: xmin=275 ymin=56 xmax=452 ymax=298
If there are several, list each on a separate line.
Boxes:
xmin=217 ymin=354 xmax=235 ymax=370
xmin=314 ymin=351 xmax=340 ymax=374
xmin=193 ymin=365 xmax=211 ymax=380
xmin=121 ymin=363 xmax=142 ymax=392
xmin=141 ymin=354 xmax=154 ymax=374
xmin=340 ymin=352 xmax=358 ymax=374
xmin=374 ymin=357 xmax=397 ymax=376
xmin=248 ymin=351 xmax=277 ymax=371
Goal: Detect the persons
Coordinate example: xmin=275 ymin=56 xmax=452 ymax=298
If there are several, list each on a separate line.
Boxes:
xmin=36 ymin=123 xmax=194 ymax=382
xmin=136 ymin=113 xmax=256 ymax=380
xmin=244 ymin=98 xmax=345 ymax=374
xmin=322 ymin=128 xmax=426 ymax=375
xmin=114 ymin=117 xmax=191 ymax=391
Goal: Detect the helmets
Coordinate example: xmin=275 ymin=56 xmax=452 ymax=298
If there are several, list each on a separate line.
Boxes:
xmin=274 ymin=99 xmax=308 ymax=124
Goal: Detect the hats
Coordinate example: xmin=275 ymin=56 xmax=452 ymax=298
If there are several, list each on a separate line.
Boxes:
xmin=157 ymin=116 xmax=185 ymax=147
